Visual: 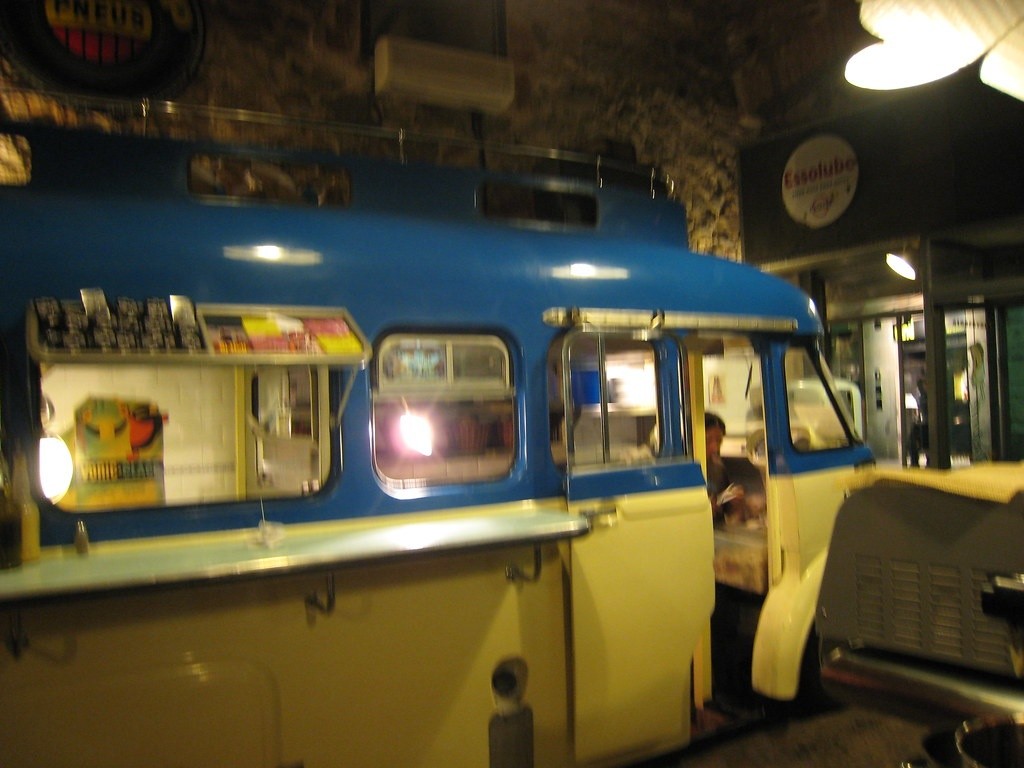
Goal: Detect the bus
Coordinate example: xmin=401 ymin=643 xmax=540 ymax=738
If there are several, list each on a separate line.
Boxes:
xmin=0 ymin=182 xmax=875 ymax=768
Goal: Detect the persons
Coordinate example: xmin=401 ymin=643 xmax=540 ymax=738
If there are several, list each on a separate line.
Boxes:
xmin=703 ymin=413 xmax=746 ymax=528
xmin=909 ymin=378 xmax=932 ymax=469
